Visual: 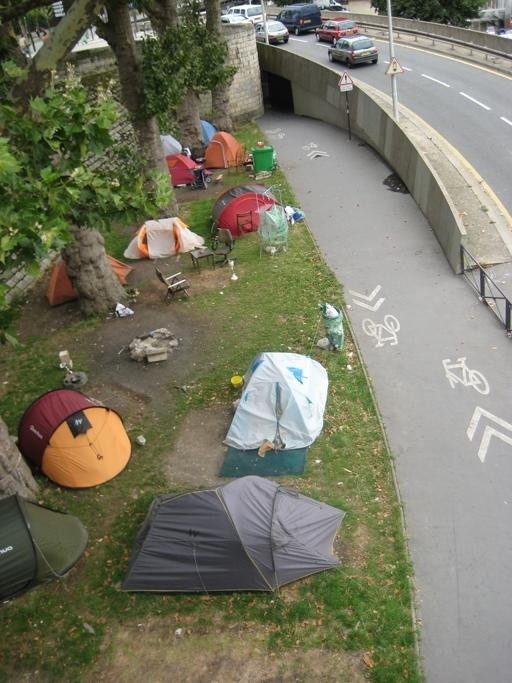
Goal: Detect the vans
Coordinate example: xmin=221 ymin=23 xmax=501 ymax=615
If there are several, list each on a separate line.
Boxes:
xmin=275 ymin=2 xmax=322 ymax=35
xmin=227 ymin=3 xmax=267 ymax=23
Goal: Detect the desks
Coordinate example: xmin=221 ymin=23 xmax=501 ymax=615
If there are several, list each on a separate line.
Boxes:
xmin=190 ymin=247 xmax=215 ymax=274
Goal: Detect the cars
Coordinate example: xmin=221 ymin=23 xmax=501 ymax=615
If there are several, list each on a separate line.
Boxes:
xmin=221 ymin=13 xmax=254 ymax=24
xmin=254 ymin=20 xmax=289 ymax=44
xmin=327 ymin=34 xmax=378 ymax=68
xmin=132 ymin=20 xmax=155 ymax=38
xmin=199 ymin=8 xmax=208 ymax=23
xmin=315 ymin=17 xmax=359 ymax=45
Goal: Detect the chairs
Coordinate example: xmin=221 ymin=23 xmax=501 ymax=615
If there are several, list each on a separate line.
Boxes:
xmin=212 ymin=226 xmax=234 ymax=267
xmin=154 ymin=264 xmax=192 ymax=297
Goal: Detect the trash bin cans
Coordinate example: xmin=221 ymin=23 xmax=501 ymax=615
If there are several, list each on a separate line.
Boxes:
xmin=251 ymin=144 xmax=274 ymax=175
xmin=319 ymin=303 xmax=345 ymax=353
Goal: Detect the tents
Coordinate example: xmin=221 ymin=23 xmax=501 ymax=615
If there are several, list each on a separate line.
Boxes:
xmin=223 ymin=352 xmax=327 ymax=451
xmin=16 ymin=388 xmax=132 ymax=490
xmin=159 ymin=134 xmax=183 ymax=154
xmin=168 ymin=153 xmax=213 ymax=184
xmin=204 ymin=131 xmax=244 ymax=169
xmin=212 ymin=182 xmax=282 ymax=236
xmin=123 ymin=216 xmax=204 ymax=260
xmin=199 ymin=119 xmax=215 ymax=147
xmin=123 ymin=474 xmax=348 ymax=591
xmin=1 ymin=495 xmax=88 ymax=602
xmin=46 ymin=253 xmax=134 ymax=307
